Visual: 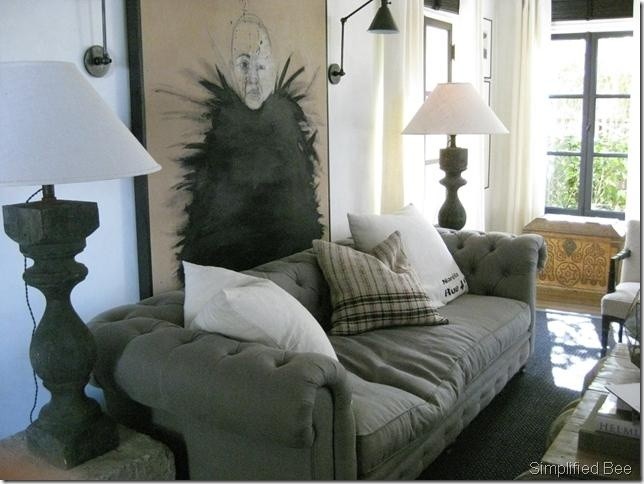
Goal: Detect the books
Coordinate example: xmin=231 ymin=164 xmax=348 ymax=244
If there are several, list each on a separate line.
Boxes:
xmin=578 ymin=382 xmax=640 ymax=461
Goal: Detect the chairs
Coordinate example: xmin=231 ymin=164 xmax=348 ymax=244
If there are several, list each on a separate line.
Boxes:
xmin=599 ymin=219 xmax=640 ymax=360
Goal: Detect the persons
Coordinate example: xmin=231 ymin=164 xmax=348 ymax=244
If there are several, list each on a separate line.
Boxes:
xmin=230 ymin=14 xmax=277 ymax=112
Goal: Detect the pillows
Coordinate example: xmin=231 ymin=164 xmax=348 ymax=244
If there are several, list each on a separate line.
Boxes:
xmin=310 ymin=200 xmax=470 ymax=336
xmin=178 ymin=260 xmax=341 ymax=367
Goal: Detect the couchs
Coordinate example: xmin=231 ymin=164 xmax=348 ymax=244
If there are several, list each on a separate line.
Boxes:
xmin=86 ymin=225 xmax=547 ymax=480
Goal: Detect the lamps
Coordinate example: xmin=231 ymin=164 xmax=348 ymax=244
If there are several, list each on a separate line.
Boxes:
xmin=328 ymin=0 xmax=400 ymax=84
xmin=0 ymin=59 xmax=163 ymax=469
xmin=398 ymin=81 xmax=510 ymax=231
